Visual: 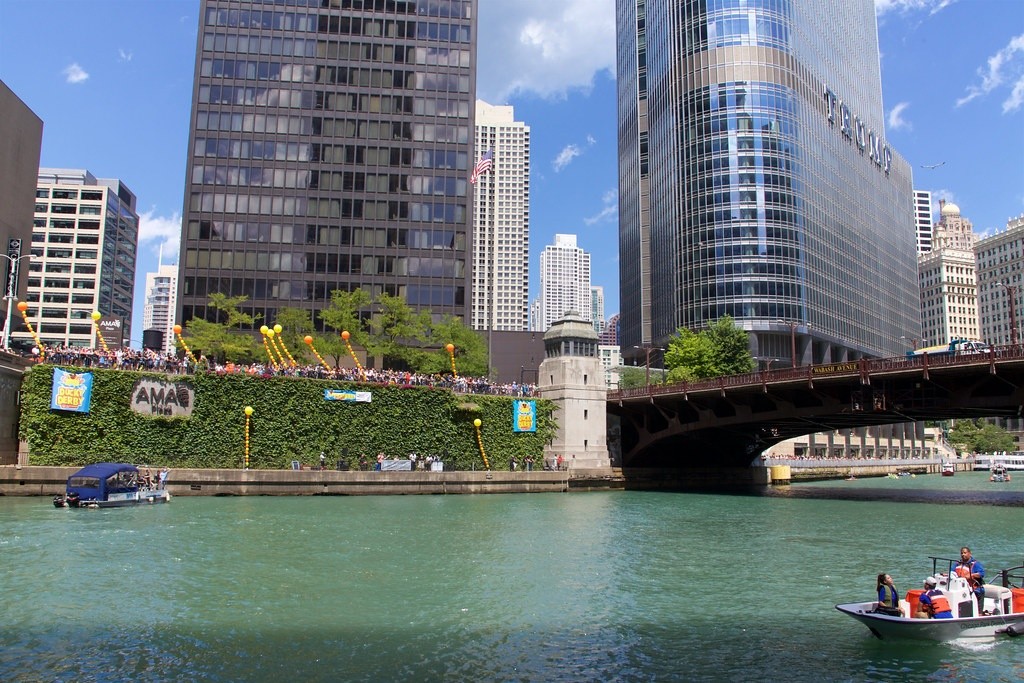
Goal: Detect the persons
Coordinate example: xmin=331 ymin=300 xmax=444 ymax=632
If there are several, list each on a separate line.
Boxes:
xmin=876 ymin=574 xmax=907 ymax=618
xmin=212 ymin=361 xmax=541 ymax=397
xmin=914 ymin=577 xmax=955 ymax=620
xmin=31 ymin=344 xmax=208 ymax=375
xmin=952 ymin=547 xmax=986 ymax=614
xmin=509 ymin=454 xmax=577 ymax=471
xmin=318 ymin=449 xmax=440 ymax=471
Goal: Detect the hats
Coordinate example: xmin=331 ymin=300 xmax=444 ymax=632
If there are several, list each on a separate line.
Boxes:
xmin=922 ymin=576 xmax=937 ymax=586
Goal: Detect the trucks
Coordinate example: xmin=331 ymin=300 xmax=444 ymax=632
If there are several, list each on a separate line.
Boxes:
xmin=905 ymin=339 xmax=996 ymax=361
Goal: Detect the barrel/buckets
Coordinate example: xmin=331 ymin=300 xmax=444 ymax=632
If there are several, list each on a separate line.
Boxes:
xmin=906 ymin=590 xmax=930 ymax=618
xmin=1010 ymin=588 xmax=1024 ymax=613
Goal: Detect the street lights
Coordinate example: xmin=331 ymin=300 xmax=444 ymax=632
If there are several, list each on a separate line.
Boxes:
xmin=996 ymin=283 xmax=1024 ymax=357
xmin=776 ymin=319 xmax=813 ymax=367
xmin=634 ymin=345 xmax=665 ymax=385
xmin=0 ymin=253 xmax=38 ymax=353
xmin=900 ymin=335 xmax=928 ymax=351
xmin=753 ymin=357 xmax=780 ymax=370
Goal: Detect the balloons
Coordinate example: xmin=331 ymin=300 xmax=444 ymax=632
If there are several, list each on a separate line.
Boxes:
xmin=91 ymin=310 xmax=109 ymax=352
xmin=446 ymin=343 xmax=457 ymax=379
xmin=260 ymin=323 xmax=297 ymax=369
xmin=244 ymin=406 xmax=253 ymax=466
xmin=304 ymin=336 xmax=333 ymax=371
xmin=17 ymin=301 xmax=45 ymax=362
xmin=173 ymin=325 xmax=198 ymax=363
xmin=473 ymin=418 xmax=489 ymax=467
xmin=340 ymin=331 xmax=364 ymax=370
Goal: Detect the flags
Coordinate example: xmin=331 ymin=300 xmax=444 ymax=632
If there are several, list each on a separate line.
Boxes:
xmin=470 ymin=148 xmax=494 ymax=183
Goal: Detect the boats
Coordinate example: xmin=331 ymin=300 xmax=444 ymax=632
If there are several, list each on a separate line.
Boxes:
xmin=941 ymin=461 xmax=954 ymax=476
xmin=836 ymin=557 xmax=1024 ymax=645
xmin=973 ymin=451 xmax=1024 ymax=471
xmin=53 ymin=462 xmax=172 ymax=509
xmin=990 ymin=464 xmax=1012 ymax=482
xmin=845 ymin=475 xmax=859 ymax=481
xmin=896 ymin=465 xmax=927 ymax=476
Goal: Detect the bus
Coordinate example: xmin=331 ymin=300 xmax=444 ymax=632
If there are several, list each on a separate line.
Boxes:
xmin=1010 ymin=451 xmax=1024 ymax=455
